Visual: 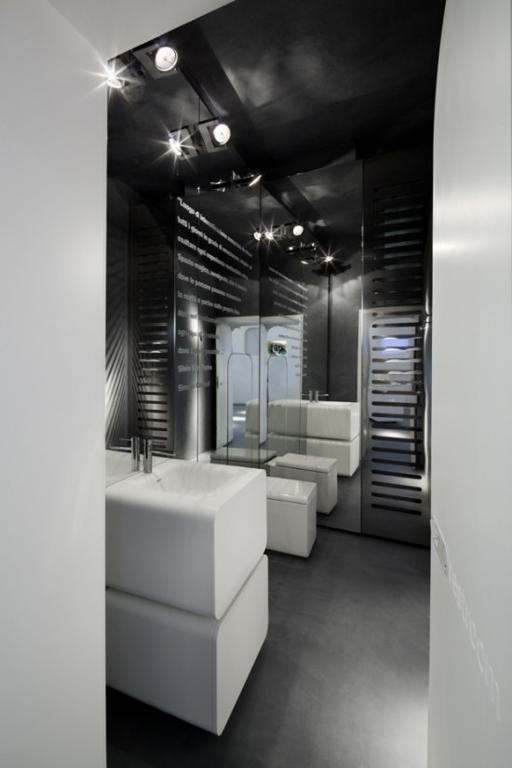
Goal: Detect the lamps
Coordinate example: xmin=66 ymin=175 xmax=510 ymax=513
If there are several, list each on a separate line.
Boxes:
xmin=196 ymin=171 xmax=263 ymax=189
xmin=260 ymin=220 xmax=304 ymax=236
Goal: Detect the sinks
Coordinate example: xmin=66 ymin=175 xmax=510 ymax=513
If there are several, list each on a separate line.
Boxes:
xmin=311 ymin=401 xmax=359 ymax=409
xmin=130 ymin=463 xmax=239 ymax=497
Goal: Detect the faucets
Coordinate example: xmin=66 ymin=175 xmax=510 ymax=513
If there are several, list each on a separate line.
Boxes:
xmin=118 ymin=435 xmax=140 ymax=471
xmin=314 ymin=390 xmax=330 ymax=400
xmin=301 ymin=392 xmax=313 ymax=401
xmin=144 ymin=439 xmax=175 ymax=472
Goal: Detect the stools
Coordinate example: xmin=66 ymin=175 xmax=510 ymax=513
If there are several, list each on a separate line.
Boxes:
xmin=269 ymin=452 xmax=337 ymax=515
xmin=265 ymin=476 xmax=317 ymax=558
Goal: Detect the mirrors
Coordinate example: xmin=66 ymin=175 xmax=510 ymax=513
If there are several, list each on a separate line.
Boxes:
xmin=105 ymin=60 xmax=176 ymax=485
xmin=258 ymin=158 xmax=365 ymax=537
